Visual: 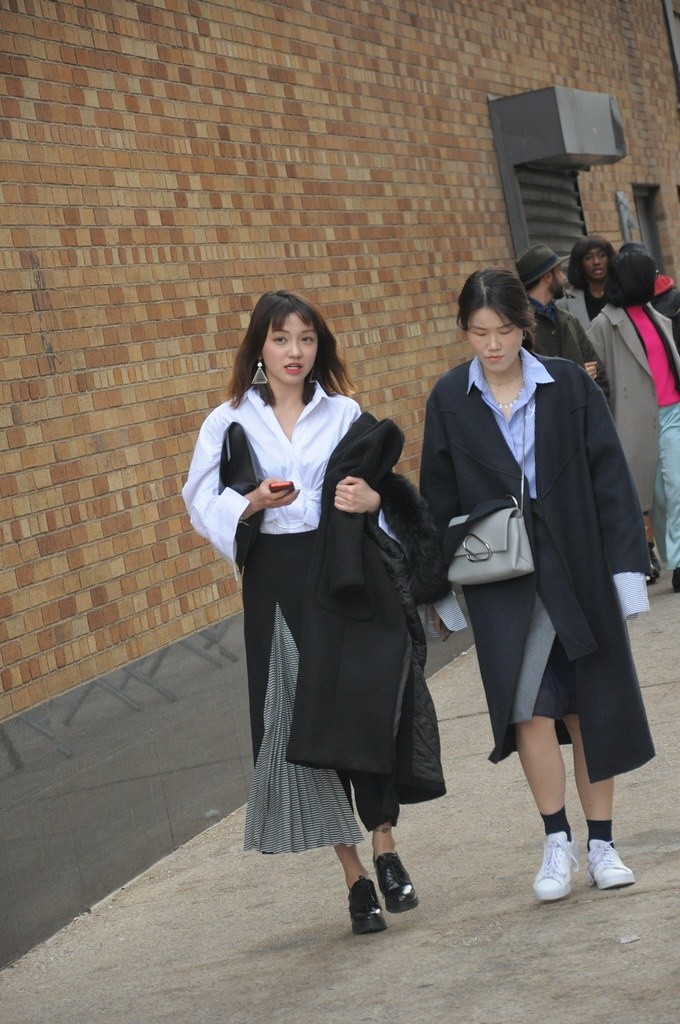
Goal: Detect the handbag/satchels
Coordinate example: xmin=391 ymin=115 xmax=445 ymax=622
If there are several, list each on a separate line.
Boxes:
xmin=446 ymin=494 xmax=536 ymax=585
xmin=219 ymin=422 xmax=264 ymax=576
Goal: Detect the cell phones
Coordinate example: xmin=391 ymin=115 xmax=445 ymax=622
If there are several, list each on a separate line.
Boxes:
xmin=439 ymin=619 xmax=453 ymax=642
xmin=269 ymin=481 xmax=295 ymax=493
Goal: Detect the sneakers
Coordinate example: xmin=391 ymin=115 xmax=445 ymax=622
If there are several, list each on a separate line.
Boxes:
xmin=534 ymin=830 xmax=580 ymax=900
xmin=585 ymin=839 xmax=637 ymax=890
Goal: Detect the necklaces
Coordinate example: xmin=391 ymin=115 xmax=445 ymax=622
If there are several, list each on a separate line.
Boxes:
xmin=496 ymin=380 xmax=524 ymax=409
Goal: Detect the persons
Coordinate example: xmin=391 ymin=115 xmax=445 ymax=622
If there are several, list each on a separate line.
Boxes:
xmin=516 ymin=245 xmax=610 ymax=403
xmin=588 ymin=252 xmax=680 ymax=594
xmin=422 ymin=270 xmax=655 ymax=901
xmin=181 ymin=288 xmax=447 ymax=934
xmin=619 ymin=243 xmax=680 ymax=357
xmin=554 ymin=237 xmax=618 ymax=330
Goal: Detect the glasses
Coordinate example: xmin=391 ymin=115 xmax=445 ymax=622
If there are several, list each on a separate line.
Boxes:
xmin=653 ymin=268 xmax=659 ymax=281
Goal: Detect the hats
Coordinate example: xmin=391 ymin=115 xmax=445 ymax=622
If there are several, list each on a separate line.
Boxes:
xmin=512 ymin=243 xmax=573 ymax=287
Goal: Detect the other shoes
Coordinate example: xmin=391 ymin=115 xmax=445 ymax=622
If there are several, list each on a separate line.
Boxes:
xmin=644 ymin=541 xmax=661 ymax=584
xmin=672 ymin=567 xmax=680 ymax=593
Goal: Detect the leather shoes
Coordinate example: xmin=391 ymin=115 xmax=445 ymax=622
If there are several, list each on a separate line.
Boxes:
xmin=371 ymin=848 xmax=419 ymax=914
xmin=348 ymin=878 xmax=388 ymax=936
